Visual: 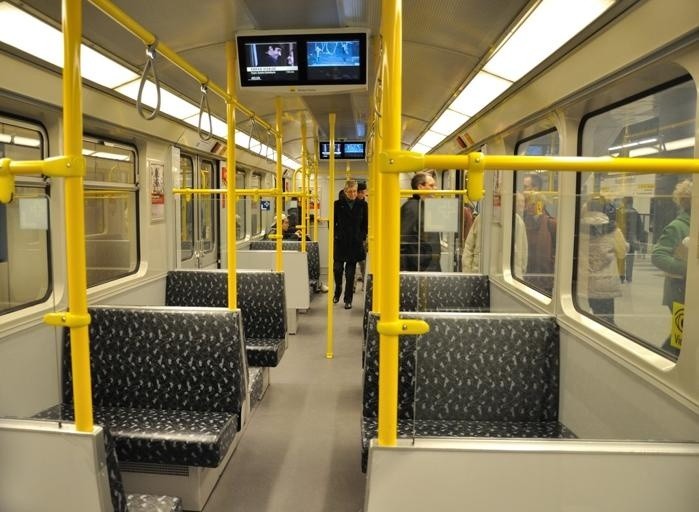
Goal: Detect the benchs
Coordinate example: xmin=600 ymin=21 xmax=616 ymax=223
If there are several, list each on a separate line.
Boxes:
xmin=360 ymin=313 xmax=581 ymax=474
xmin=165 ymin=271 xmax=288 ymax=367
xmin=361 ymin=272 xmax=490 ymax=374
xmin=0 ymin=416 xmax=183 ymax=512
xmin=250 ymin=241 xmax=319 ymax=292
xmin=32 ymin=308 xmax=244 ymax=469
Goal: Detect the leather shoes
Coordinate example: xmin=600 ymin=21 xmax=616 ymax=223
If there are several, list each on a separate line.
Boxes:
xmin=333 ymin=296 xmax=339 ymax=303
xmin=345 ymin=302 xmax=352 ymax=309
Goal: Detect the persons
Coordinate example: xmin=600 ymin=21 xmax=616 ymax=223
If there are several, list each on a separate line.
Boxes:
xmin=328 ymin=180 xmax=368 ymax=309
xmin=650 ymin=179 xmax=698 ymax=350
xmin=524 ymin=193 xmax=557 ymax=293
xmin=356 ymin=182 xmax=370 ymax=202
xmin=574 ymin=194 xmax=632 ymax=326
xmin=251 ymin=43 xmax=288 ymax=65
xmin=462 ymin=187 xmax=529 ymax=275
xmin=398 ymin=173 xmax=443 ymax=272
xmin=519 ymin=174 xmax=553 ymax=198
xmin=621 ymin=196 xmax=646 ymax=281
xmin=263 ymin=213 xmax=331 ymax=296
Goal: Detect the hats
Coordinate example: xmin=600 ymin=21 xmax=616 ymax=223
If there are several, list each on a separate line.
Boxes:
xmin=271 ymin=214 xmax=286 ymax=228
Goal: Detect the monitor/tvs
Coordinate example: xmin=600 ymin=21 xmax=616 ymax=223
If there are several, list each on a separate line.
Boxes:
xmin=318 ymin=139 xmax=369 ymax=162
xmin=524 ymin=143 xmax=547 ymax=156
xmin=235 ymin=28 xmax=370 ymax=95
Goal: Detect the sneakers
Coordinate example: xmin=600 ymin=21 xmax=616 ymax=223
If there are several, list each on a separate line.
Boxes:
xmin=319 ymin=284 xmax=328 ymax=292
